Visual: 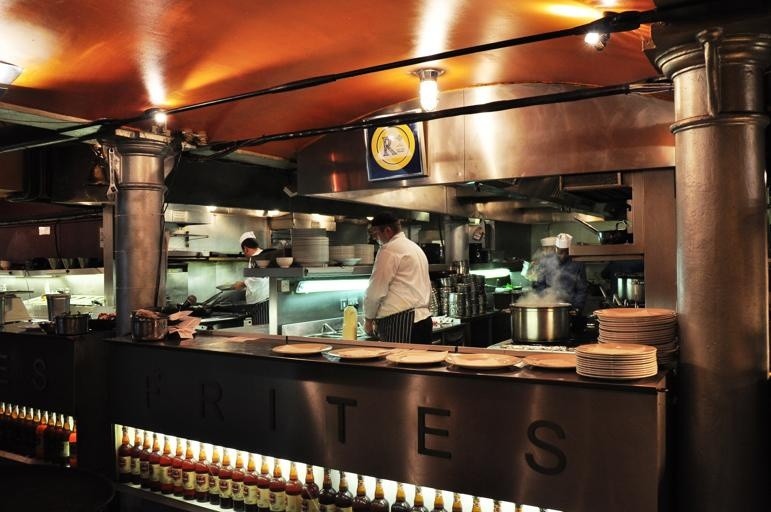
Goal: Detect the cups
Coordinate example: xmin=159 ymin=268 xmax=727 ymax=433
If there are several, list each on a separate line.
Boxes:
xmin=48 ymin=257 xmax=89 ymax=268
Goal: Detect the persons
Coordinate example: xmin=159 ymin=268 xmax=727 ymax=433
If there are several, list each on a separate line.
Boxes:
xmin=531 ymin=232 xmax=589 ymax=336
xmin=363 ymin=212 xmax=434 ymax=344
xmin=231 ymin=231 xmax=269 ymax=326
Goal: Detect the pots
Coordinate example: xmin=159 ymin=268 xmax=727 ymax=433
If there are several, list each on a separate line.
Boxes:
xmin=507 ymin=301 xmax=574 ymax=342
xmin=574 ymin=216 xmax=628 ymax=245
xmin=613 ymin=271 xmax=645 ymax=304
xmin=430 ymin=260 xmax=489 ymax=318
xmin=51 ymin=313 xmax=89 ymax=336
xmin=131 ymin=311 xmax=169 ymax=341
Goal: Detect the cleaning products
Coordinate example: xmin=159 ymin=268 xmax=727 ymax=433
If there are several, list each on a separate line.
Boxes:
xmin=342 ymin=298 xmax=358 ymax=340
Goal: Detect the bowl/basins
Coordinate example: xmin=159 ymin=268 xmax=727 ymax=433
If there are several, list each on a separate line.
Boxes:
xmin=88 ymin=319 xmax=116 ymax=330
xmin=255 ymin=259 xmax=271 ymax=268
xmin=276 ymin=256 xmax=294 ymax=269
xmin=0 ymin=261 xmax=11 ymax=270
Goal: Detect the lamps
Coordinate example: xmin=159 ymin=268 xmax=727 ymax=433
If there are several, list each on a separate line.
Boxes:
xmin=584 ymin=29 xmax=611 ymax=52
xmin=410 ymin=66 xmax=446 ymax=112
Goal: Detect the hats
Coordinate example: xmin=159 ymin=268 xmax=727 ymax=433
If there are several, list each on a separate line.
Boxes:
xmin=373 ymin=211 xmax=400 ymax=227
xmin=554 ymin=233 xmax=573 ymax=249
xmin=540 ymin=237 xmax=559 ymax=247
xmin=240 ymin=231 xmax=257 ymax=247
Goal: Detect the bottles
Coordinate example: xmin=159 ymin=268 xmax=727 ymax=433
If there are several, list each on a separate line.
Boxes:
xmin=342 ymin=306 xmax=358 ymax=341
xmin=0 ymin=400 xmax=77 ymax=471
xmin=115 ymin=425 xmax=549 ymax=512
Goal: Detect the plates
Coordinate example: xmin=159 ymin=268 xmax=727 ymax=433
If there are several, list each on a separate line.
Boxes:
xmin=216 ymin=284 xmax=234 ymax=290
xmin=385 ymin=350 xmax=450 ymax=364
xmin=329 ymin=244 xmax=375 ymax=267
xmin=292 ymin=236 xmax=329 ymax=264
xmin=592 ymin=307 xmax=680 ymax=360
xmin=18 ymin=325 xmax=41 ymax=330
xmin=327 ymin=346 xmax=386 ymax=359
xmin=270 ymin=343 xmax=333 ymax=354
xmin=523 ymin=351 xmax=580 ymax=369
xmin=574 ymin=343 xmax=660 ymax=379
xmin=444 ymin=352 xmax=522 ymax=370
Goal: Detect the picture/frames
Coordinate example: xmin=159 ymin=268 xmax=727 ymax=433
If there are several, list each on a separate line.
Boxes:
xmin=363 ymin=109 xmax=428 ymax=181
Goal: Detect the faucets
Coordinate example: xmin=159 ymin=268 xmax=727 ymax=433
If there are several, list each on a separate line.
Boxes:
xmin=320 ymin=321 xmax=337 ymax=334
